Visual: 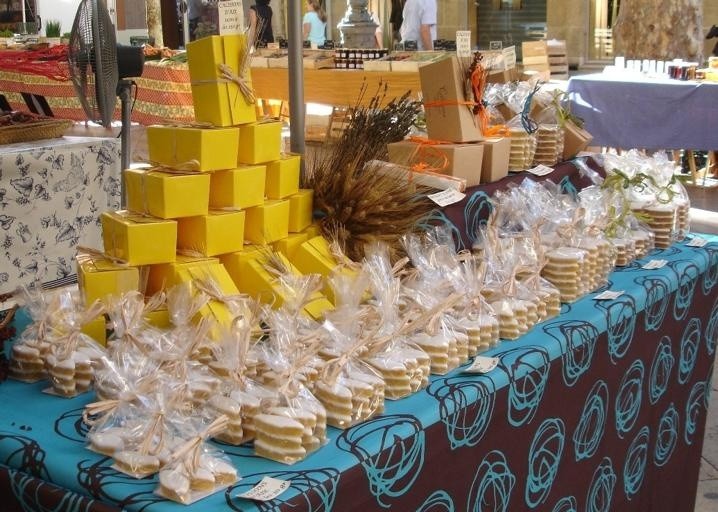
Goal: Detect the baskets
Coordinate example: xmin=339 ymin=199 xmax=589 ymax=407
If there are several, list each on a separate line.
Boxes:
xmin=0 ymin=119 xmax=73 ymax=144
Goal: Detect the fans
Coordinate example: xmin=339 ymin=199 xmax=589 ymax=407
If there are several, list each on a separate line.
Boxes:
xmin=66 ymin=1 xmax=145 ymax=211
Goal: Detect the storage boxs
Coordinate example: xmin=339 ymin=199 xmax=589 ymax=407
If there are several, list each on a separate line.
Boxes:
xmin=418 ymin=56 xmax=486 ymax=143
xmin=145 ymin=34 xmax=282 ymax=173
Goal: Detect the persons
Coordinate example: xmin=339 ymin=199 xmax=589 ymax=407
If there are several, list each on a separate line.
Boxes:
xmin=247 ymin=0 xmax=274 ymax=49
xmin=302 ymin=0 xmax=327 ymax=47
xmin=399 ymin=0 xmax=437 ymax=51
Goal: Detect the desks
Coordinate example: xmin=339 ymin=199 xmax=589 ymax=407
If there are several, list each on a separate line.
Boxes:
xmin=569 ymin=71 xmax=718 ymax=187
xmin=3 ymin=138 xmax=717 ymax=511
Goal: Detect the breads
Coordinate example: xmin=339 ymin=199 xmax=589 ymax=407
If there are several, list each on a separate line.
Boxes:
xmin=396 ymin=225 xmax=499 ymax=376
xmin=12 ymin=282 xmax=106 ymax=396
xmin=85 ymin=280 xmax=242 ymax=506
xmin=504 ymin=78 xmax=565 ymax=173
xmin=511 ymin=176 xmax=657 ymax=302
xmin=209 ymin=240 xmax=430 ymax=466
xmin=602 ymin=148 xmax=688 ymax=248
xmin=475 ymin=224 xmax=561 ymax=340
